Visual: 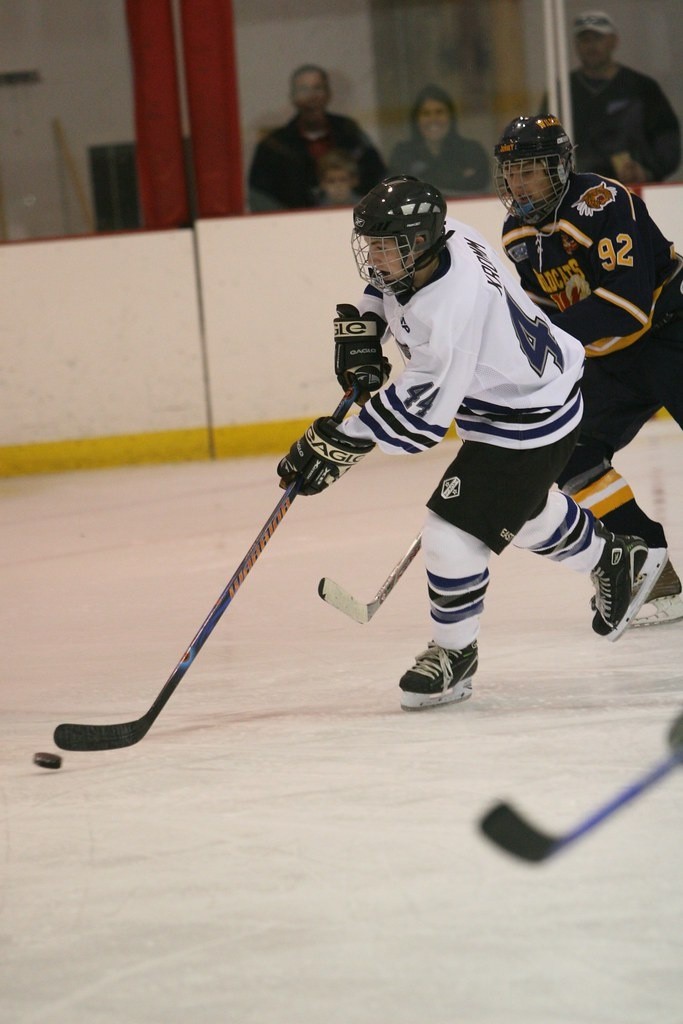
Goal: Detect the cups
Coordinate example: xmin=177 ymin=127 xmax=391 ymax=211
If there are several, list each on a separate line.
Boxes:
xmin=611 ymin=152 xmax=632 ymax=182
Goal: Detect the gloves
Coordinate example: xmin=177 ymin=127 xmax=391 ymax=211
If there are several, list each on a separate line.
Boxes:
xmin=276 ymin=417 xmax=377 ymax=495
xmin=335 ymin=304 xmax=392 ymax=406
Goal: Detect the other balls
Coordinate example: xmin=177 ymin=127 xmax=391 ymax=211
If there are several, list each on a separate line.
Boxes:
xmin=33 ymin=750 xmax=63 ymax=771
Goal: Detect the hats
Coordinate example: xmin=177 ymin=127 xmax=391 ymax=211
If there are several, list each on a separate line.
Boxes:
xmin=571 ymin=12 xmax=619 ymax=39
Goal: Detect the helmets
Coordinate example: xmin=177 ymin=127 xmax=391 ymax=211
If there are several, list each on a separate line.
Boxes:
xmin=351 ymin=174 xmax=455 ymax=296
xmin=494 ymin=113 xmax=574 ymax=226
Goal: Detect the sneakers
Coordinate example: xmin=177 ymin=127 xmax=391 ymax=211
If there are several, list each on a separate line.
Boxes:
xmin=399 ymin=639 xmax=479 ymax=712
xmin=590 ymin=515 xmax=670 ymax=644
xmin=633 ymin=557 xmax=683 ymax=625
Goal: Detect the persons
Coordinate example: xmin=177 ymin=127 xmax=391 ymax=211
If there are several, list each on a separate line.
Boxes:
xmin=277 ymin=114 xmax=683 ymax=695
xmin=391 ymin=83 xmax=491 ymax=192
xmin=246 ymin=64 xmax=388 ymax=213
xmin=537 ymin=10 xmax=683 ymax=184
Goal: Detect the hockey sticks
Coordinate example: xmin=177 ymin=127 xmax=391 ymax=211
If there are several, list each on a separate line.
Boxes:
xmin=476 ymin=749 xmax=682 ymax=866
xmin=50 ymin=378 xmax=360 ymax=753
xmin=316 ymin=528 xmax=426 ymax=626
